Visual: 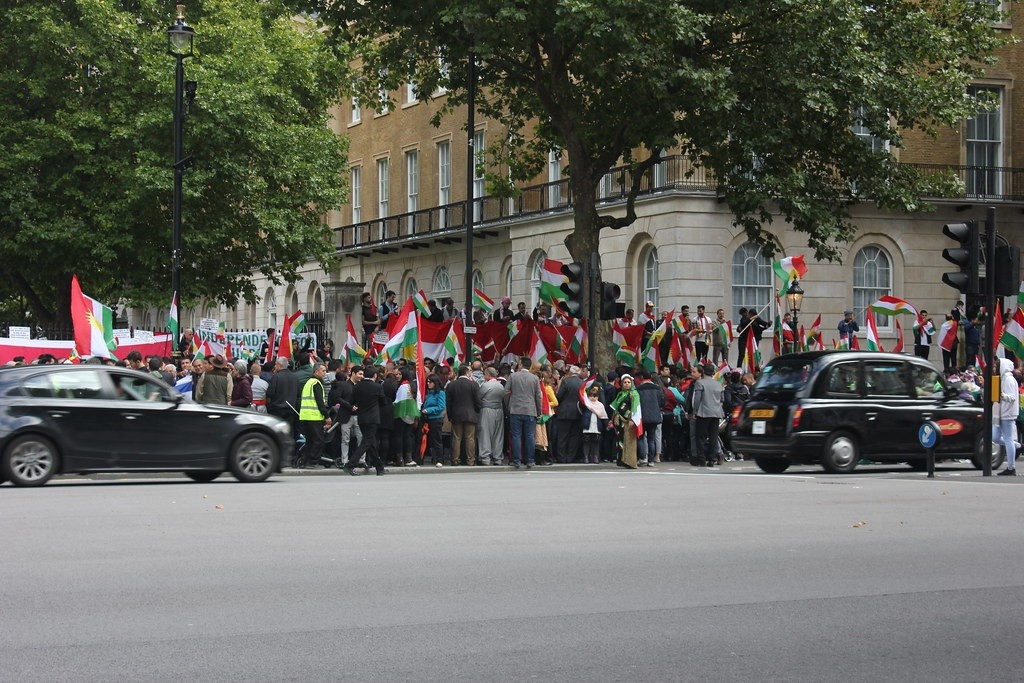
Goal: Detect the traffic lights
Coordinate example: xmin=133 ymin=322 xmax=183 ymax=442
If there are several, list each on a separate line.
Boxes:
xmin=560 ymin=262 xmax=585 ymax=319
xmin=602 ymin=281 xmax=627 ymax=320
xmin=942 ymin=221 xmax=979 ymax=297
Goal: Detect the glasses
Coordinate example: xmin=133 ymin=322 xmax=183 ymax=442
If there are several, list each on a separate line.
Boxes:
xmin=427 ymin=381 xmax=432 ymax=383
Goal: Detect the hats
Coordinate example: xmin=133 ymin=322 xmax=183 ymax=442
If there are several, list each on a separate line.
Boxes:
xmin=646 ymin=301 xmax=655 ymax=307
xmin=209 ymin=354 xmax=228 ymax=368
xmin=607 ymin=372 xmax=617 ymax=379
xmin=498 ymin=366 xmax=514 ymax=374
xmin=501 ymin=297 xmax=512 ymax=304
xmin=844 ymin=311 xmax=852 ymax=315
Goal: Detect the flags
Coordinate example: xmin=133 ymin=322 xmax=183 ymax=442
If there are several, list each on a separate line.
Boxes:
xmin=70 ymin=274 xmax=115 ymax=357
xmin=166 ymin=254 xmax=1024 ymax=424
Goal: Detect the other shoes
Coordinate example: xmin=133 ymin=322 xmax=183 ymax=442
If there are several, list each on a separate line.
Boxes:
xmin=1015 ymin=443 xmax=1024 ymax=459
xmin=997 ymin=467 xmax=1017 ymax=475
xmin=300 ymin=454 xmax=744 ymax=474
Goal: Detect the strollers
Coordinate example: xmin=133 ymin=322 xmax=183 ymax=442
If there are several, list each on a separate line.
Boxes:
xmin=295 ymin=404 xmax=344 ymax=469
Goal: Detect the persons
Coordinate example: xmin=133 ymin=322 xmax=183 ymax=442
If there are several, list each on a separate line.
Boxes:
xmin=991 ymin=357 xmax=1024 ymax=475
xmin=913 ymin=309 xmax=936 ymax=360
xmin=7 ymin=289 xmax=796 ymax=477
xmin=837 ymin=310 xmax=859 ymax=349
xmin=942 ymin=300 xmax=1024 ymax=460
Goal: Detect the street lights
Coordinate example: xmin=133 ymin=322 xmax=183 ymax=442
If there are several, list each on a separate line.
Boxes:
xmin=786 ymin=274 xmax=805 ymax=352
xmin=167 ymin=5 xmax=192 ymax=372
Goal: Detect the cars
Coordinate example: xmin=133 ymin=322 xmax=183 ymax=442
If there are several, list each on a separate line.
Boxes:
xmin=0 ymin=364 xmax=294 ymax=488
xmin=727 ymin=350 xmax=1004 ymax=474
